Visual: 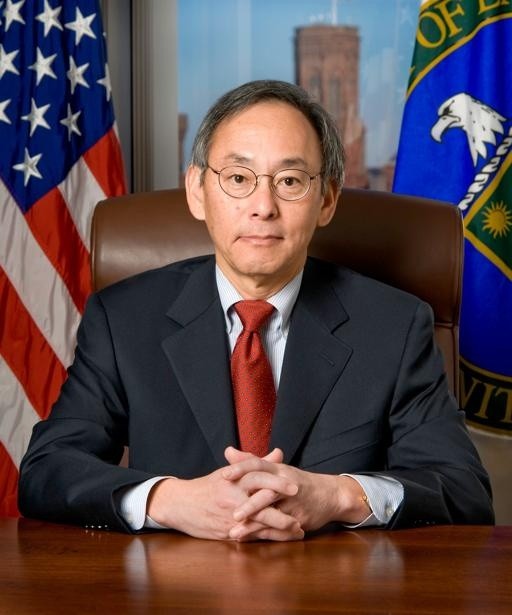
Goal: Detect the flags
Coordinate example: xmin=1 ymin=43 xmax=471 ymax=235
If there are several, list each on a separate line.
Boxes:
xmin=0 ymin=1 xmax=127 ymax=518
xmin=392 ymin=0 xmax=512 ymax=525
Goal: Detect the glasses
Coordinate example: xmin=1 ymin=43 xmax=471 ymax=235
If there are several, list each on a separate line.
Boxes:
xmin=204 ymin=158 xmax=328 ymax=202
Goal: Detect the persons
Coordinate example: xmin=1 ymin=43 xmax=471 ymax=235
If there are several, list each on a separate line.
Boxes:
xmin=18 ymin=77 xmax=495 ymax=545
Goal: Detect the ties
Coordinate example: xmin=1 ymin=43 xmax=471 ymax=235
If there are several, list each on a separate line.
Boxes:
xmin=222 ymin=296 xmax=281 ymax=464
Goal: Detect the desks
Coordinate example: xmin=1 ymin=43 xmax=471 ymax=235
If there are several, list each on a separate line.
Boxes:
xmin=0 ymin=517 xmax=512 ymax=614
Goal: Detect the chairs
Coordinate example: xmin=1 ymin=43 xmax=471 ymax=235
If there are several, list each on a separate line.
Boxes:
xmin=91 ymin=186 xmax=465 ymax=411
